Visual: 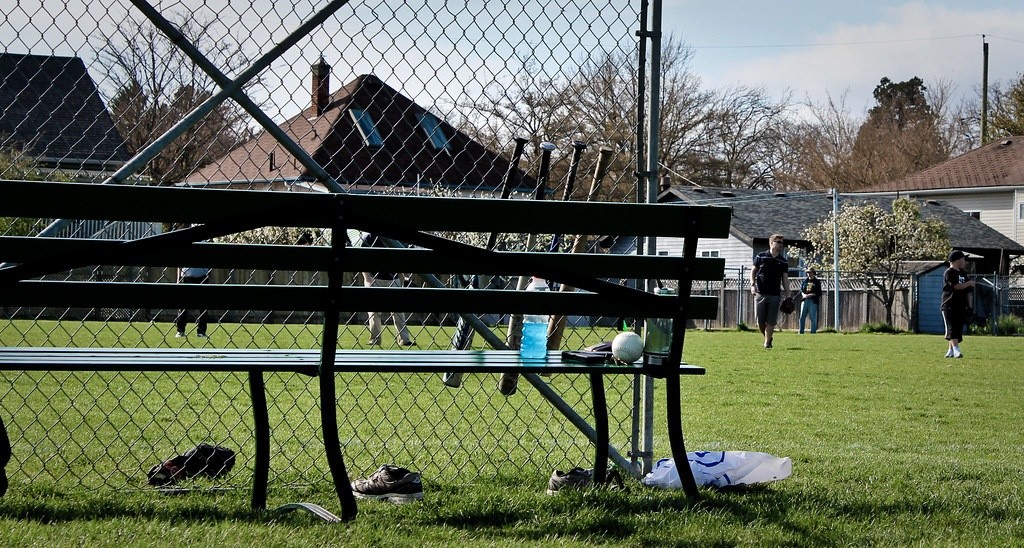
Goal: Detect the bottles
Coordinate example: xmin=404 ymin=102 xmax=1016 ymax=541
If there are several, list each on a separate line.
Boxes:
xmin=520 ymin=276 xmax=551 ymax=358
xmin=643 ymin=287 xmax=676 ymax=366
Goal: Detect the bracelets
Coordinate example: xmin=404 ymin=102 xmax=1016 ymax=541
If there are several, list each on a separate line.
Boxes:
xmin=751 ymin=282 xmax=754 ymax=286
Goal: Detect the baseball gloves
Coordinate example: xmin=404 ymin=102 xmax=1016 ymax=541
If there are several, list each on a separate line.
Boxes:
xmin=779 ymin=297 xmax=796 ymax=313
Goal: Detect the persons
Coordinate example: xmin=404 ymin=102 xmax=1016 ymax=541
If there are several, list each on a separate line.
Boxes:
xmin=174 ymin=225 xmax=208 ymax=338
xmin=940 ymin=251 xmax=976 ymax=360
xmin=800 ymin=268 xmax=821 ymax=334
xmin=361 ymin=233 xmax=418 ymax=347
xmin=749 ymin=235 xmax=794 ymax=349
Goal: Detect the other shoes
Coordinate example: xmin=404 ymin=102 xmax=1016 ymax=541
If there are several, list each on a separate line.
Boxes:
xmin=367 ymin=340 xmax=381 ymax=345
xmin=197 ymin=334 xmax=206 ymax=337
xmin=404 ymin=341 xmax=417 ymax=345
xmin=176 ymin=332 xmax=187 ymax=338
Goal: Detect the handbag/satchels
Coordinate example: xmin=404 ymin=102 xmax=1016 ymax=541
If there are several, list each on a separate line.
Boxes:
xmin=639 ymin=450 xmax=793 ymax=490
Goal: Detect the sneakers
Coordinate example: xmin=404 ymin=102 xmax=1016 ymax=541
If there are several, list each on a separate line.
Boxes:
xmin=148 ymin=441 xmax=234 ymax=485
xmin=954 ymin=349 xmax=963 ymax=358
xmin=944 ymin=349 xmax=953 ymax=358
xmin=350 ymin=463 xmax=424 ymax=501
xmin=547 ymin=468 xmax=592 ymax=496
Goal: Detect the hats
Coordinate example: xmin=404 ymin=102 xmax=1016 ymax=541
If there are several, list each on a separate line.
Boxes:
xmin=805 ymin=267 xmax=816 ymax=273
xmin=947 ymin=249 xmax=971 ymax=262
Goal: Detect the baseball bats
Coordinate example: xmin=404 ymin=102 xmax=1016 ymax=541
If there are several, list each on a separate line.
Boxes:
xmin=499 ymin=141 xmax=555 ymax=396
xmin=544 ymin=140 xmax=587 ymax=292
xmin=536 ymin=146 xmax=612 ymax=378
xmin=440 ymin=136 xmax=529 ymax=389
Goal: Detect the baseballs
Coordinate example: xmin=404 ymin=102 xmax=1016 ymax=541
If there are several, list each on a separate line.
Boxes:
xmin=612 ymin=331 xmax=644 ymax=364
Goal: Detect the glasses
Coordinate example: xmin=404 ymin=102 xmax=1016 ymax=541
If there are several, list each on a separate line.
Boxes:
xmin=773 ymin=240 xmax=784 ymax=244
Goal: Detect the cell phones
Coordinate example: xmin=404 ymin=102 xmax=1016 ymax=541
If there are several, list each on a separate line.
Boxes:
xmin=561 ymin=350 xmax=605 ymax=365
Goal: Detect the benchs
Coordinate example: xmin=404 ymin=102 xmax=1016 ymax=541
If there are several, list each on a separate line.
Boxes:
xmin=0 ymin=173 xmax=734 ymax=505
